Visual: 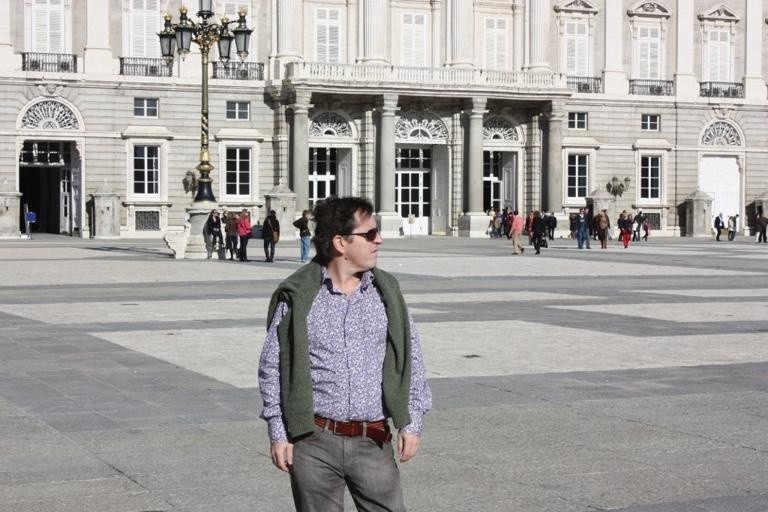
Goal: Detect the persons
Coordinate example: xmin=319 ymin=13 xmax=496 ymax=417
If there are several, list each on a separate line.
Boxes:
xmin=228 ymin=209 xmax=240 ymax=260
xmin=238 ymin=207 xmax=253 ymax=260
xmin=258 ymin=208 xmax=284 ymax=263
xmin=291 ymin=208 xmax=310 ymax=262
xmin=485 ymin=205 xmax=768 ymax=257
xmin=256 ymin=195 xmax=433 ymax=512
xmin=207 ymin=209 xmax=225 ymax=250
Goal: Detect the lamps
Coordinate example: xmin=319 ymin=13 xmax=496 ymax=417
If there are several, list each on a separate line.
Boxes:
xmin=607 ymin=176 xmax=631 ymax=198
xmin=184 ymin=170 xmax=199 ymax=192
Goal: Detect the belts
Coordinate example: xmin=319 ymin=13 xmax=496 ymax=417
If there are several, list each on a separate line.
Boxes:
xmin=314 ymin=415 xmax=389 ymax=443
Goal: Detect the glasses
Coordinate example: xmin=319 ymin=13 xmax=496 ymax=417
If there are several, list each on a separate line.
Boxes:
xmin=345 ymin=226 xmax=378 ymax=242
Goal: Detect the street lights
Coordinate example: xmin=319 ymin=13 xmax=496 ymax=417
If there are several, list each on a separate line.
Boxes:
xmin=182 ymin=170 xmax=199 ymax=202
xmin=155 ymin=1 xmax=256 ymax=203
xmin=606 ymin=175 xmax=631 ymax=227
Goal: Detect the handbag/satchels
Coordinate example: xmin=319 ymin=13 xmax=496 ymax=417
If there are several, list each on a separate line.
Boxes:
xmin=540 ymin=240 xmax=547 ymax=247
xmin=273 ymin=232 xmax=278 ymax=242
xmin=247 ymin=233 xmax=252 ymax=238
